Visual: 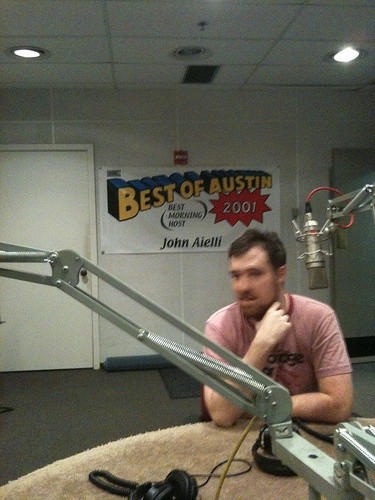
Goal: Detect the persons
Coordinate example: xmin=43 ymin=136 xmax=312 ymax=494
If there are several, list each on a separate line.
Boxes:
xmin=203 ymin=229 xmax=354 ymax=429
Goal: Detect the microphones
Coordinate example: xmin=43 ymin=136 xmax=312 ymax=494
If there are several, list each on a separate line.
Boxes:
xmin=304 ymin=202 xmax=328 ymax=290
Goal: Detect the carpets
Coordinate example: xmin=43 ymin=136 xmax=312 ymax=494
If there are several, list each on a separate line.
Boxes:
xmin=159 ymin=367 xmax=202 ymax=399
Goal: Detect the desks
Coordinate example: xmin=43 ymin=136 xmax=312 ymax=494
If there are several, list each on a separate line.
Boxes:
xmin=0 ymin=418 xmax=375 ymax=500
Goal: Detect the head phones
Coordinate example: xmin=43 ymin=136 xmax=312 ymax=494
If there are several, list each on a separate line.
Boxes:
xmin=251 ymin=424 xmax=296 ymax=476
xmin=129 ymin=468 xmax=198 ymax=500
